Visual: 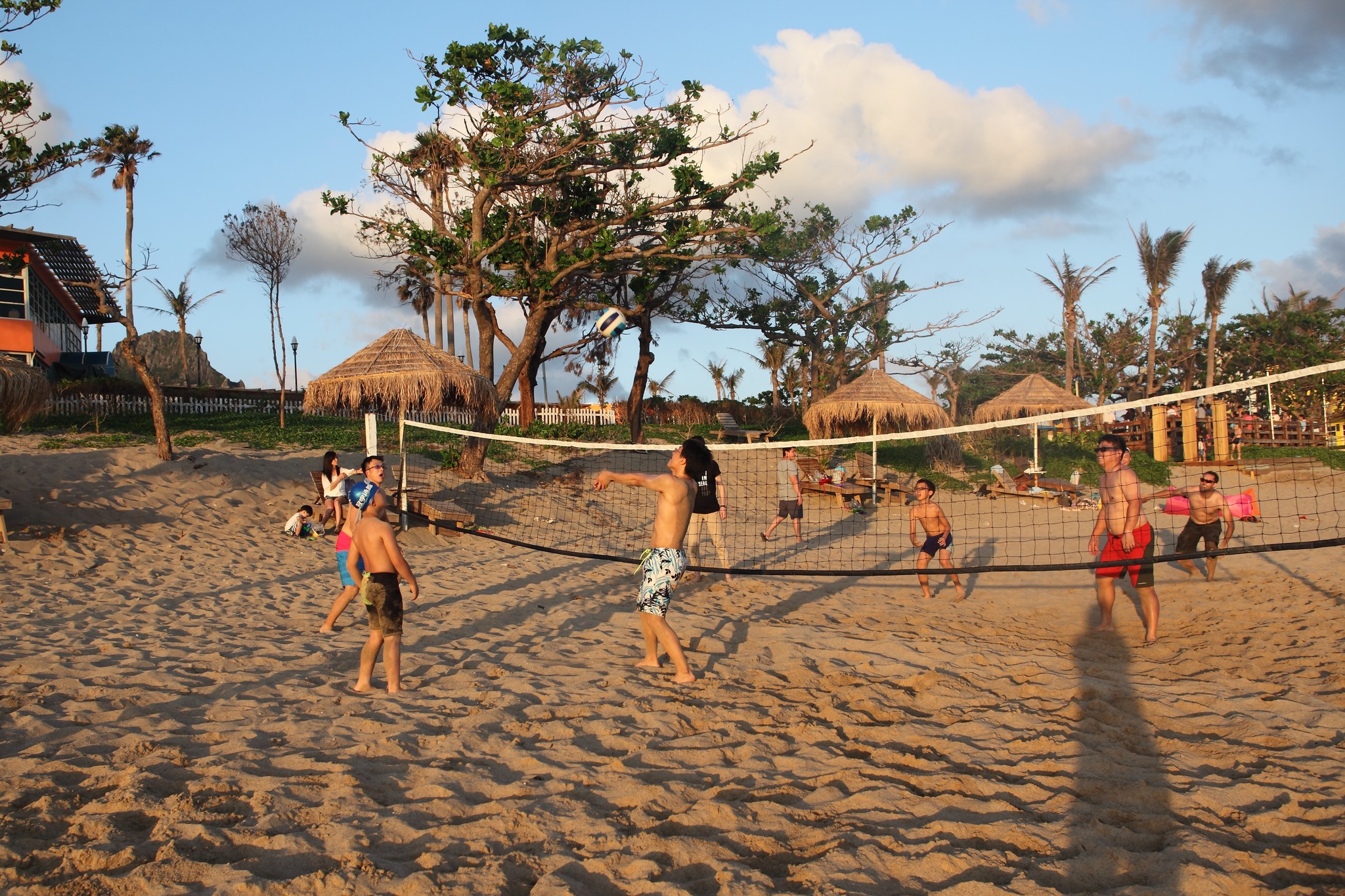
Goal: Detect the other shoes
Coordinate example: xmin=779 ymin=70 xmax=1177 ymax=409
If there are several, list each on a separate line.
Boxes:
xmin=1030 ymin=504 xmax=1039 ymax=507
xmin=976 ymin=492 xmax=983 ymax=496
xmin=990 ymin=496 xmax=997 ymax=500
xmin=1018 ymin=500 xmax=1027 ymax=505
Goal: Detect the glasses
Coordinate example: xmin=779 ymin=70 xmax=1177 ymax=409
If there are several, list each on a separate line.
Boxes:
xmin=674 ymin=448 xmax=687 ymax=461
xmin=1200 ymin=478 xmax=1216 ymax=483
xmin=914 ymin=487 xmax=931 ymax=491
xmin=1094 ymin=447 xmax=1120 ymax=453
xmin=365 ymin=465 xmax=387 ymax=472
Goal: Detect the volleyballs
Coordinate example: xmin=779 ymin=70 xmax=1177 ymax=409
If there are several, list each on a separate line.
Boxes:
xmin=596 ymin=308 xmax=627 ymax=337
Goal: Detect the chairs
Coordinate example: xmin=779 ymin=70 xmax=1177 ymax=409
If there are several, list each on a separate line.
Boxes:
xmin=985 ymin=456 xmax=1086 ymax=505
xmin=795 ymin=450 xmax=916 ymax=508
xmin=305 ymin=464 xmax=477 ymax=536
xmin=708 ymin=412 xmax=774 ymax=445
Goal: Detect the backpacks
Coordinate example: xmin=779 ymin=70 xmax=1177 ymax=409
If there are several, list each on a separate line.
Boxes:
xmin=1016 ymin=483 xmax=1028 ymax=491
xmin=979 ymin=484 xmax=992 ymax=496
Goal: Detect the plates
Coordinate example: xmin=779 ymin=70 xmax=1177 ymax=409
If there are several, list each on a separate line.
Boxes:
xmin=1040 ymin=491 xmax=1051 ymax=494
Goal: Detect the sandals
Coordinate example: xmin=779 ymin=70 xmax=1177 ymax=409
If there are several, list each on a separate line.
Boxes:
xmin=292 ymin=534 xmax=302 ymax=539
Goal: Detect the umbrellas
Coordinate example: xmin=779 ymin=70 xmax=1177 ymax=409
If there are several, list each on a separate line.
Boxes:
xmin=300 ymin=328 xmax=501 ymax=534
xmin=975 ymin=374 xmax=1103 ymax=487
xmin=804 ymin=369 xmax=951 ymax=504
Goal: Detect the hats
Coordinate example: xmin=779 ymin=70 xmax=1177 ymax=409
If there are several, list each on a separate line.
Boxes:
xmin=348 ymin=478 xmax=379 ymax=511
xmin=1230 ymin=423 xmax=1237 ymax=429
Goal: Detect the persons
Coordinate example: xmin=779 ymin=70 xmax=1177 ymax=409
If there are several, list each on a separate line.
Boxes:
xmin=592 ymin=439 xmax=713 ymax=684
xmin=687 ymin=436 xmax=733 ymax=582
xmin=1102 ymin=400 xmax=1309 ymax=462
xmin=1088 ymin=433 xmax=1160 ymax=641
xmin=348 ymin=478 xmax=419 ymax=694
xmin=285 ymin=505 xmax=313 ymax=539
xmin=909 ymin=479 xmax=965 ymax=599
xmin=320 ymin=451 xmax=364 ymax=532
xmin=1140 ymin=471 xmax=1235 ymax=582
xmin=318 ymin=456 xmax=404 ymax=633
xmin=763 ymin=447 xmax=808 ymax=543
xmin=1120 ymin=447 xmax=1150 ymax=504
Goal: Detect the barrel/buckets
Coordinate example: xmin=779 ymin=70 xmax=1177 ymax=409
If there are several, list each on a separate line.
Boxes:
xmin=311 ymin=524 xmax=325 ymax=538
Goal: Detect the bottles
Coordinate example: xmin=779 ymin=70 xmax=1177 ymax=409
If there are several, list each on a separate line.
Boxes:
xmin=808 ymin=475 xmax=815 ymax=483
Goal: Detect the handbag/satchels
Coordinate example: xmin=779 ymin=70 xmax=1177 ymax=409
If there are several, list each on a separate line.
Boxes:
xmin=1076 ymin=499 xmax=1098 ymax=511
xmin=1058 ymin=497 xmax=1072 ymax=507
xmin=1070 ymin=471 xmax=1080 ymax=485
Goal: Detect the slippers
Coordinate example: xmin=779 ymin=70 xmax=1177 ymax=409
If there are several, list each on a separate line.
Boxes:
xmin=760 ymin=532 xmax=769 ymax=542
xmin=333 ymin=529 xmax=340 ymax=532
xmin=804 ymin=540 xmax=810 ymax=543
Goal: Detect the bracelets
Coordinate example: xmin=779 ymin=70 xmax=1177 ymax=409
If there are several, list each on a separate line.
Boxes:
xmin=356 ymin=470 xmax=359 ymax=474
xmin=720 ymin=505 xmax=725 ymax=508
